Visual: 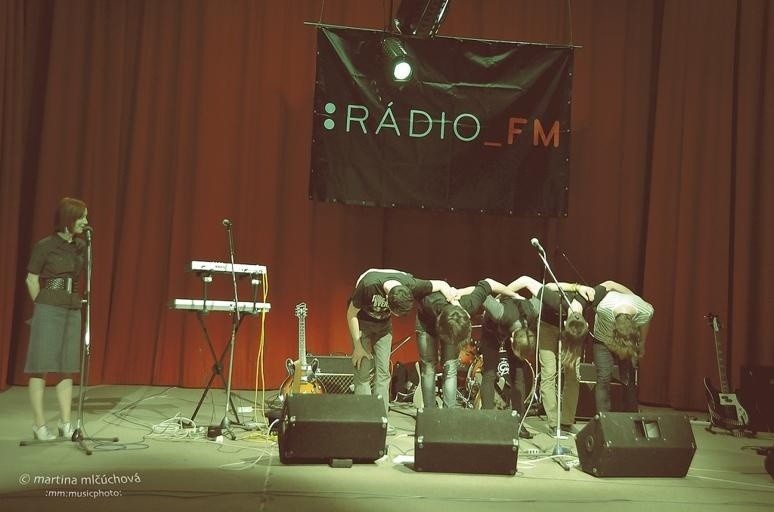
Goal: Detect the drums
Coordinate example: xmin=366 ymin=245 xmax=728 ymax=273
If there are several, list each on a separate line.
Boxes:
xmin=436 ymin=339 xmax=482 ymax=388
xmin=465 ymin=353 xmax=536 ymax=405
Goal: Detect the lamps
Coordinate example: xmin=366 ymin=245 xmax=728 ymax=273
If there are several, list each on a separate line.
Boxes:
xmin=380 ymin=13 xmax=416 ymax=84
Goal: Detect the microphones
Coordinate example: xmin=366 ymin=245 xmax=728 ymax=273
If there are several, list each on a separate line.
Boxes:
xmin=530 ymin=236 xmax=545 ymax=253
xmin=83 ymin=223 xmax=94 ymax=246
xmin=221 ymin=217 xmax=233 ymax=228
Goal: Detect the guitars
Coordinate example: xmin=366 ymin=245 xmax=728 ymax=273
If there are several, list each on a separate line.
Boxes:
xmin=279 ymin=302 xmax=328 ymax=406
xmin=703 ymin=313 xmax=752 ymax=430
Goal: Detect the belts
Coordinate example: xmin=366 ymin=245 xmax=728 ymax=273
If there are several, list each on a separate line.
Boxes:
xmin=43 ymin=277 xmax=80 ymax=294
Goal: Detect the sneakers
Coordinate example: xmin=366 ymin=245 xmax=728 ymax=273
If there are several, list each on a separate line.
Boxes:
xmin=560 ymin=422 xmax=581 ymax=436
xmin=548 ymin=426 xmax=567 ymax=442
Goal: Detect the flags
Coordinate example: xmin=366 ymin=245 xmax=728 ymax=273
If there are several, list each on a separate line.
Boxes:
xmin=308 ymin=24 xmax=573 ymax=219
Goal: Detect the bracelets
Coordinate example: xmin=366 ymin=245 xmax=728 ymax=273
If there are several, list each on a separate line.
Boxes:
xmin=572 ymin=283 xmax=580 ymax=292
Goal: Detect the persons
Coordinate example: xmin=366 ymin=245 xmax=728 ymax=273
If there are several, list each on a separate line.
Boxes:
xmin=594 ymin=290 xmax=654 ymax=413
xmin=355 ymin=268 xmax=526 ymax=409
xmin=348 ymin=268 xmax=470 ymax=432
xmin=495 ymin=274 xmax=635 ymax=438
xmin=447 ymin=282 xmax=595 ymax=438
xmin=26 ymin=198 xmax=90 ymax=441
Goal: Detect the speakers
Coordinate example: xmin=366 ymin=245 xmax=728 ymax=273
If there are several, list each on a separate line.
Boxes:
xmin=277 ymin=394 xmax=389 ymax=469
xmin=415 ymin=409 xmax=520 ymax=475
xmin=306 ymin=355 xmax=376 ymax=393
xmin=579 ymin=362 xmax=637 ymax=419
xmin=575 ymin=412 xmax=697 ymax=478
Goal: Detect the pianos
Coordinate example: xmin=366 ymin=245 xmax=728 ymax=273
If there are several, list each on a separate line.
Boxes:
xmin=166 ymin=299 xmax=272 ymax=313
xmin=187 ymin=260 xmax=268 ymax=276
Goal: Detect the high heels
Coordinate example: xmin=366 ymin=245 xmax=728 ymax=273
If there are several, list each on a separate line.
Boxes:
xmin=57 ymin=422 xmax=76 ymax=439
xmin=31 ymin=424 xmax=58 ymax=441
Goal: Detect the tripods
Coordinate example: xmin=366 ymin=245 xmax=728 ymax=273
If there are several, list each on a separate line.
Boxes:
xmin=522 ymin=328 xmax=581 ymax=472
xmin=18 ymin=290 xmax=120 ymax=455
xmin=201 ymin=322 xmax=262 ymax=439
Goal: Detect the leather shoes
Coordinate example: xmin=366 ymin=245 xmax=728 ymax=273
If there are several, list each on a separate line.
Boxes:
xmin=517 ymin=420 xmax=533 ymax=439
